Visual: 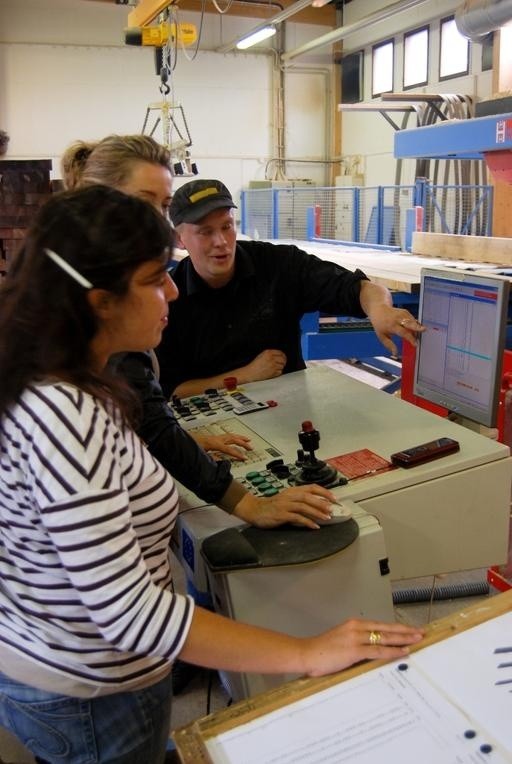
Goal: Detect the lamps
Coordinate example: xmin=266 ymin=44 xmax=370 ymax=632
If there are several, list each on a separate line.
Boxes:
xmin=234 ymin=24 xmax=278 ymax=52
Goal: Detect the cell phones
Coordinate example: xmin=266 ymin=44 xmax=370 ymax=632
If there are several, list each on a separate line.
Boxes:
xmin=390 ymin=436 xmax=461 ymax=469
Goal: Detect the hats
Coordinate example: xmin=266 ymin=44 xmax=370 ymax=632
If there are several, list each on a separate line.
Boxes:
xmin=169 ymin=179 xmax=237 ymax=228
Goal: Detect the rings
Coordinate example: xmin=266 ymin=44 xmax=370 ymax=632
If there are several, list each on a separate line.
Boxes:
xmin=368 ymin=631 xmax=380 ymax=644
xmin=401 ymin=317 xmax=413 ymax=326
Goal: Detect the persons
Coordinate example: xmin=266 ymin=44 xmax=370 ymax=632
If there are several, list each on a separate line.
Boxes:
xmin=149 ymin=175 xmax=430 ymax=403
xmin=59 ymin=134 xmax=339 ymax=698
xmin=0 ymin=182 xmax=430 ymax=763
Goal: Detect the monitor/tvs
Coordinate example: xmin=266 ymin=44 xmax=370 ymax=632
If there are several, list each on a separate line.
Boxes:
xmin=413 ymin=267 xmax=510 ymax=434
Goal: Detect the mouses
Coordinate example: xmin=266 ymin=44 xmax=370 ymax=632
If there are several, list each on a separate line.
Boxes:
xmin=313 ymin=501 xmax=353 ymax=526
